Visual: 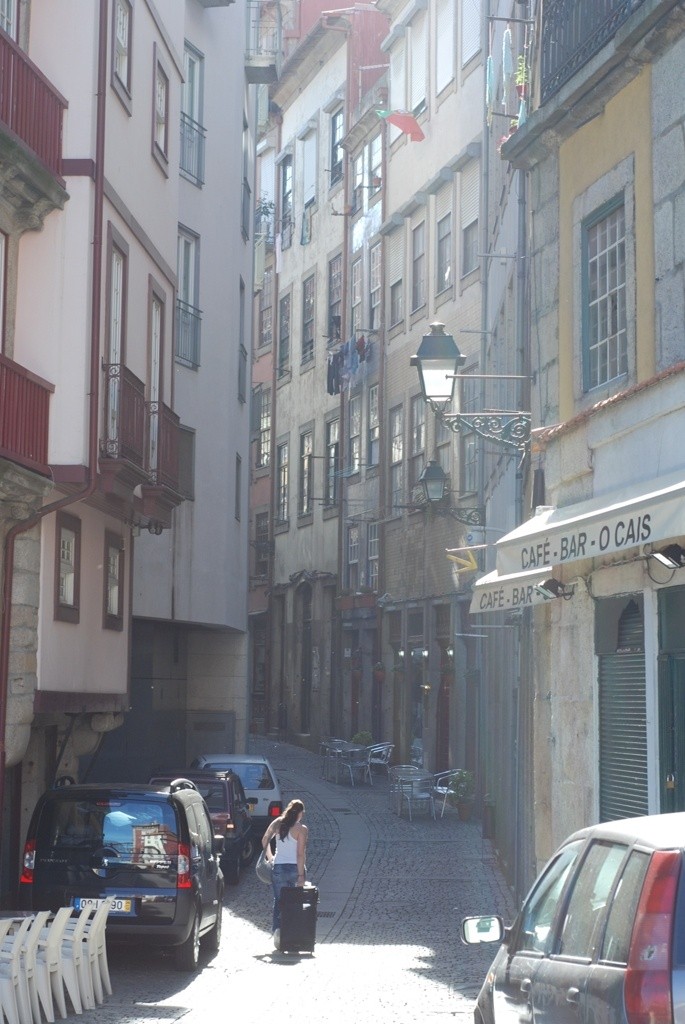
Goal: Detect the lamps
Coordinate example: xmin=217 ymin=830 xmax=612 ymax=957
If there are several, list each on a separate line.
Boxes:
xmin=534 ymin=577 xmax=575 ymax=601
xmin=651 ymin=543 xmax=685 ymax=569
xmin=419 ymin=461 xmax=486 ymax=526
xmin=410 ymin=321 xmax=537 ymax=451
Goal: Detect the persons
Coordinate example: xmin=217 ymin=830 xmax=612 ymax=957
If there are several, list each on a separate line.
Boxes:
xmin=262 ymin=799 xmax=308 ymax=954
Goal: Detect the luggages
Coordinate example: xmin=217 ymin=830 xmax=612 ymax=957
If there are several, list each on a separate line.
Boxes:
xmin=278 ymin=884 xmax=319 ymax=955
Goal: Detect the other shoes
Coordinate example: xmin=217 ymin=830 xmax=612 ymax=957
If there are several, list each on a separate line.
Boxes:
xmin=273 ymin=929 xmax=281 ymax=951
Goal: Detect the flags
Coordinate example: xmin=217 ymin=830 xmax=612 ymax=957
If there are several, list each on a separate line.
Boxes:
xmin=376 ymin=108 xmax=426 ymax=142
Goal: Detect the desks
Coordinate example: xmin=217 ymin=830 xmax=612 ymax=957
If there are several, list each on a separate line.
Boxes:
xmin=331 ymin=742 xmax=365 ymax=782
xmin=390 ymin=770 xmax=434 ymax=817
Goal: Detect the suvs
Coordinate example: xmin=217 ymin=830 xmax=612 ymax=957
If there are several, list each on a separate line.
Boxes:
xmin=20 ymin=776 xmax=227 ymax=972
xmin=190 ymin=753 xmax=282 ymax=825
xmin=146 ymin=768 xmax=255 ymax=881
xmin=463 ymin=814 xmax=685 ymax=1024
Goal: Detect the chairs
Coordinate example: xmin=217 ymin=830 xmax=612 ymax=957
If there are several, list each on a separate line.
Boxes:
xmin=320 ymin=736 xmax=461 ymax=824
xmin=0 ymin=894 xmax=116 ymax=1024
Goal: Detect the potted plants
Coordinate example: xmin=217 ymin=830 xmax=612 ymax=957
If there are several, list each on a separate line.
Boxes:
xmin=333 ymin=589 xmax=354 ymax=610
xmin=354 ymin=585 xmax=377 ymax=607
xmin=450 ymin=770 xmax=476 ymax=819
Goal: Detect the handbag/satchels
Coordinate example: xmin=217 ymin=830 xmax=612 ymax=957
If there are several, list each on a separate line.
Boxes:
xmin=255 ymin=835 xmax=273 ymax=884
